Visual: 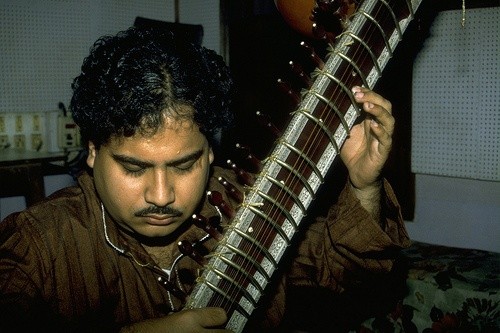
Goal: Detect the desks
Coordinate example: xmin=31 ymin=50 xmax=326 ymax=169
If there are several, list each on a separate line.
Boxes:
xmin=313 ymin=241 xmax=500 ymax=333
xmin=0 ymin=146 xmax=69 ymax=209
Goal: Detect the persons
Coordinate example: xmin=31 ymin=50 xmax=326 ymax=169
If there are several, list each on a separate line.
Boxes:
xmin=0 ymin=24 xmax=411 ymax=333
